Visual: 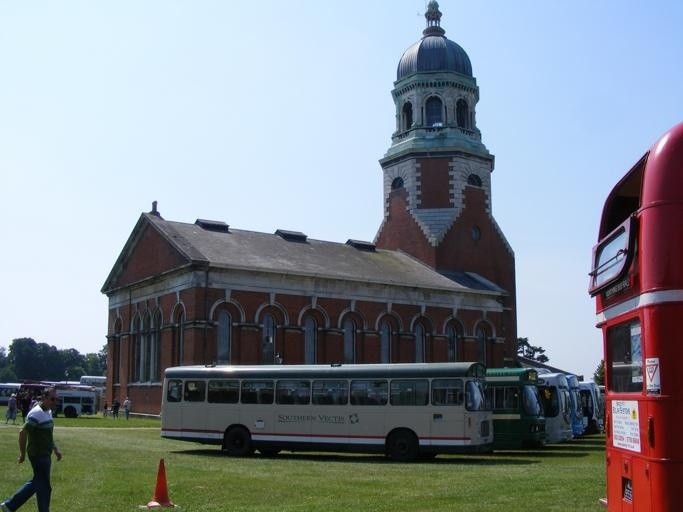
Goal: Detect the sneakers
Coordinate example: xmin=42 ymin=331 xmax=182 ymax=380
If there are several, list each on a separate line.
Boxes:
xmin=0 ymin=502 xmax=10 ymax=512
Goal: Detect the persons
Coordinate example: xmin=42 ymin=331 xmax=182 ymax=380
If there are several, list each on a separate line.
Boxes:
xmin=1 ymin=386 xmax=63 ymax=512
xmin=110 ymin=396 xmax=122 ymax=420
xmin=122 ymin=394 xmax=132 ymax=421
xmin=102 ymin=399 xmax=108 ymax=416
xmin=4 ymin=385 xmax=58 ymax=425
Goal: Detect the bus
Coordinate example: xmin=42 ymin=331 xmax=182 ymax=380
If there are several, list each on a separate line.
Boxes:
xmin=482 ymin=367 xmax=546 ymax=450
xmin=159 ymin=356 xmax=494 ymax=462
xmin=531 ymin=369 xmax=605 ymax=444
xmin=17 ymin=383 xmax=97 ymax=419
xmin=0 ymin=375 xmax=106 ymax=409
xmin=587 ymin=118 xmax=682 ymax=512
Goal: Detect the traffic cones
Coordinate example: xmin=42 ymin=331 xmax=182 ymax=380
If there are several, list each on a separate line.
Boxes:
xmin=138 ymin=457 xmax=180 ymax=510
